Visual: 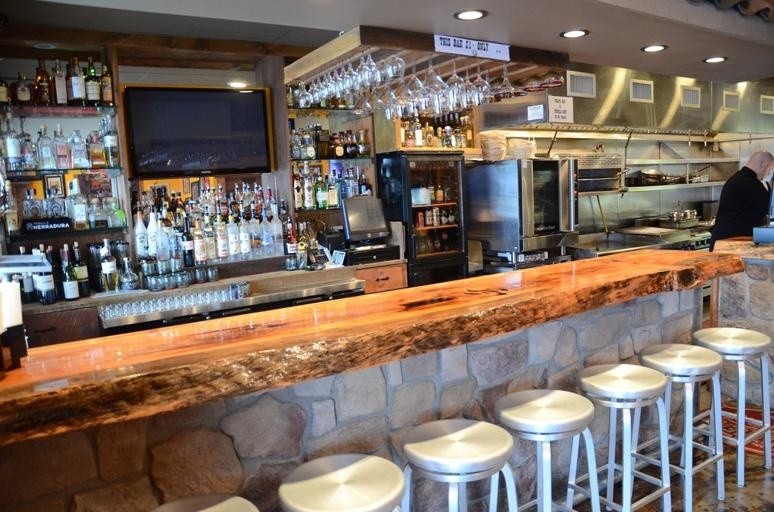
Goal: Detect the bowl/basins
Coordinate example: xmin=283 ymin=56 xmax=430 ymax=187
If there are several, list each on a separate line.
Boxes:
xmin=481 ymin=134 xmax=537 ymax=162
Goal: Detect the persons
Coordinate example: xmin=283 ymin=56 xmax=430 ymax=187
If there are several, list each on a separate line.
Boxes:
xmin=709 ymin=150 xmax=774 ymax=252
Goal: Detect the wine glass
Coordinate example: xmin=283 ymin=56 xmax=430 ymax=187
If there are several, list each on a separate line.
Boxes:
xmin=285 ymin=47 xmax=564 ymax=122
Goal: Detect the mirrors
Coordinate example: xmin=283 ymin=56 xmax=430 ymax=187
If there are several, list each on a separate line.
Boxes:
xmin=0 ymin=57 xmax=126 ymax=231
xmin=130 ymin=173 xmax=264 ymax=227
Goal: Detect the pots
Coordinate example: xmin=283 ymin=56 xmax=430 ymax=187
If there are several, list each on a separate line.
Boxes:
xmin=670 ymin=209 xmax=696 ymax=221
xmin=625 ymin=169 xmax=683 ymax=186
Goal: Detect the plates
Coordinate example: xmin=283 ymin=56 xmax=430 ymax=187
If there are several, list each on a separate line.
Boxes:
xmin=534 ymin=171 xmax=553 ymax=182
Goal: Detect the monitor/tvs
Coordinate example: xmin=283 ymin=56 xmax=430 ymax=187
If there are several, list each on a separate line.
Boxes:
xmin=125 ymin=86 xmax=271 ymax=180
xmin=342 ymin=199 xmax=390 ymax=242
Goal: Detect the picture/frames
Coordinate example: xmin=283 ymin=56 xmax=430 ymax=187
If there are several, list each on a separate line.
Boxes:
xmin=42 ymin=172 xmax=67 ymax=199
xmin=181 ymin=177 xmax=190 ymax=196
xmin=189 ymin=181 xmax=200 ymax=201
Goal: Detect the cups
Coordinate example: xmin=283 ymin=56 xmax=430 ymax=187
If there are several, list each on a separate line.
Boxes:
xmin=98 ymin=281 xmax=252 ymax=324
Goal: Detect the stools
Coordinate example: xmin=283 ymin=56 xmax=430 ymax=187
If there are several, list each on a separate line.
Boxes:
xmin=491 ymin=386 xmax=604 ymax=510
xmin=144 ymin=492 xmax=264 ymax=511
xmin=680 ymin=325 xmax=774 ymax=487
xmin=396 ymin=417 xmax=524 ymax=511
xmin=566 ymin=359 xmax=676 ymax=511
xmin=629 ymin=342 xmax=728 ymax=511
xmin=273 ymin=451 xmax=410 ymax=511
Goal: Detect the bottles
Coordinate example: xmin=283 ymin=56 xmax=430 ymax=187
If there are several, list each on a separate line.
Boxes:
xmin=129 ymin=122 xmax=372 ymax=291
xmin=412 ymin=181 xmax=457 ymax=253
xmin=400 ymin=109 xmax=480 ymax=148
xmin=0 ymin=53 xmax=138 ymax=306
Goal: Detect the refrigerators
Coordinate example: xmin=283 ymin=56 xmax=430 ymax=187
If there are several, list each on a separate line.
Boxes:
xmin=373 ymin=149 xmax=469 ymax=284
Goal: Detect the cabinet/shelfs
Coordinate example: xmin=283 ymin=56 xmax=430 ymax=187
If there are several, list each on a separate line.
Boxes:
xmin=477 ymin=127 xmax=742 ymax=195
xmin=0 ymin=101 xmax=128 ymax=242
xmin=394 ymin=102 xmax=483 ymax=157
